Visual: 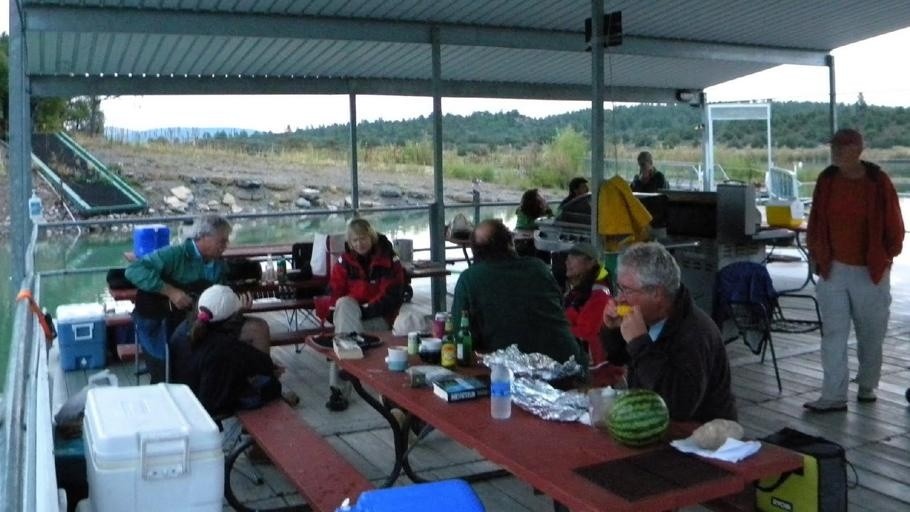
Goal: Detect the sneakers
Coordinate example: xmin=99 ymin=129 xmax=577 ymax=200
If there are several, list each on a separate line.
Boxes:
xmin=326 ymin=386 xmax=348 ymax=410
xmin=803 ymin=398 xmax=847 ymax=412
xmin=858 ymin=387 xmax=876 ymax=402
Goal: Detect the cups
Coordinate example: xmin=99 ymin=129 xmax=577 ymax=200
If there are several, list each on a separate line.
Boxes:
xmin=408 ymin=331 xmax=420 ymax=355
xmin=418 ymin=337 xmax=441 ymax=363
xmin=432 ymin=320 xmax=445 ymax=338
xmin=385 ymin=346 xmax=408 ymax=371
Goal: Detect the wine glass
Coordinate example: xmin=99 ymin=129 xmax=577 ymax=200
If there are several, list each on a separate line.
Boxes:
xmin=313 ymin=295 xmax=331 ymax=339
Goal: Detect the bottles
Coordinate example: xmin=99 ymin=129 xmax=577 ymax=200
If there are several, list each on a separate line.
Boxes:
xmin=491 ymin=357 xmax=511 ymax=419
xmin=457 ymin=311 xmax=472 ymax=367
xmin=266 ymin=254 xmax=274 ymax=281
xmin=441 ymin=317 xmax=456 ymax=370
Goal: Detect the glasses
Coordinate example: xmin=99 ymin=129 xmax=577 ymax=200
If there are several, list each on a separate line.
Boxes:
xmin=615 ymin=282 xmax=642 ymax=297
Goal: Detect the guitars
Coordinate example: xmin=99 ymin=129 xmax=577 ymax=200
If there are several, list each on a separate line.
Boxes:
xmin=137 ymin=277 xmax=298 ymax=318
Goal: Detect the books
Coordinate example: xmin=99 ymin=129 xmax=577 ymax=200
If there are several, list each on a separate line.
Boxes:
xmin=430 ymin=372 xmax=492 ymax=402
xmin=331 ymin=336 xmax=365 ymax=360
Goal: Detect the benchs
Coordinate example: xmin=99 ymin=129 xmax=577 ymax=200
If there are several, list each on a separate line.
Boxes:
xmin=103 ymin=299 xmax=314 ymax=326
xmin=705 ymin=478 xmax=761 ymax=512
xmin=114 ymin=327 xmax=402 ymax=358
xmin=224 ymin=402 xmax=375 ymax=509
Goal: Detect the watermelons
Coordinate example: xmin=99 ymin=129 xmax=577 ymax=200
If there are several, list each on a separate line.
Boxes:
xmin=604 ymin=390 xmax=670 ymax=448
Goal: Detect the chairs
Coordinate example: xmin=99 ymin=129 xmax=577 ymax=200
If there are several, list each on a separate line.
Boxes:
xmin=720 ymin=263 xmax=821 ymax=393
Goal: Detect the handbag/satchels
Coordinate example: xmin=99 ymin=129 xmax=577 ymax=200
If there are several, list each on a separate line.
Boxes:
xmin=754 ymin=427 xmax=858 ymax=511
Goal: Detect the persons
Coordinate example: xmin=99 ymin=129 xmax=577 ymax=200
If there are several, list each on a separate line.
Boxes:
xmin=598 ymin=243 xmax=739 ymax=423
xmin=558 ymin=176 xmax=591 ymax=215
xmin=513 ymin=188 xmax=552 ymax=266
xmin=125 ymin=213 xmax=298 ymax=404
xmin=629 ymin=151 xmax=669 ymax=193
xmin=802 ymin=130 xmax=905 ymax=412
xmin=326 ymin=219 xmax=403 ymax=411
xmin=172 ymin=285 xmax=281 ymax=463
xmin=451 ymin=219 xmax=588 ymax=372
xmin=551 ymin=241 xmax=628 ymax=391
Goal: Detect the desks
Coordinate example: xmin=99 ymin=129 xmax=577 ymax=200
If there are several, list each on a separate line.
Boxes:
xmin=305 ymin=332 xmax=803 ymax=512
xmin=125 ymin=244 xmax=299 ymax=258
xmin=445 ymin=230 xmax=540 ymax=264
xmin=284 ymin=269 xmax=451 ymax=353
xmin=757 ymin=221 xmax=821 ymax=293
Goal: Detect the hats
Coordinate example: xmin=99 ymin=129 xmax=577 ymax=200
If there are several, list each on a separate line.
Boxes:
xmin=197 ymin=284 xmax=241 ymax=323
xmin=392 ymin=312 xmax=426 ymax=336
xmin=561 ymin=243 xmax=599 ymax=258
xmin=826 ymin=129 xmax=862 ymax=144
